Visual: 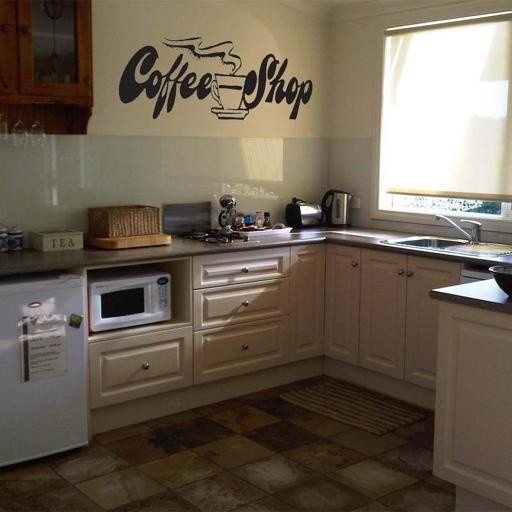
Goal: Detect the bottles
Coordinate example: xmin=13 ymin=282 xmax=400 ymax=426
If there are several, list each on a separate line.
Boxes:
xmin=255 ymin=212 xmax=272 ymax=228
xmin=0 ymin=225 xmax=24 ymax=253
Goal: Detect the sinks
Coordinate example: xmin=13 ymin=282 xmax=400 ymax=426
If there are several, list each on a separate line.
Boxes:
xmin=379 ymin=236 xmax=469 ymax=249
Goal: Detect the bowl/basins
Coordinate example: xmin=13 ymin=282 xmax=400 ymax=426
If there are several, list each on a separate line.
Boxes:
xmin=488 ymin=264 xmax=512 ymax=298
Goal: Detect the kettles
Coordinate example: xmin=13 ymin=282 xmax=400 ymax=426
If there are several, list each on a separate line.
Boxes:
xmin=322 ymin=189 xmax=353 ymax=229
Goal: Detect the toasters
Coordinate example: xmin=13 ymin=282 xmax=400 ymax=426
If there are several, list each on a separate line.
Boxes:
xmin=285 ymin=203 xmax=323 ymax=228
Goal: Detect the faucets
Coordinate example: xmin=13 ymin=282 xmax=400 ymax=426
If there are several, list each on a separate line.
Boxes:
xmin=435 ymin=214 xmax=483 ymax=245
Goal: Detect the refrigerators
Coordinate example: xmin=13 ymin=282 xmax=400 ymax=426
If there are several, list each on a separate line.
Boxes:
xmin=0 ymin=273 xmax=89 ymax=467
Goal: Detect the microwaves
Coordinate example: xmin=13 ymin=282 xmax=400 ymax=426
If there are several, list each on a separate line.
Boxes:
xmin=88 ymin=267 xmax=173 ymax=335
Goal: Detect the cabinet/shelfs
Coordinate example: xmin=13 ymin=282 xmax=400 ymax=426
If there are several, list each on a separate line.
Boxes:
xmin=359 ymin=242 xmax=464 ymax=413
xmin=1 ymin=0 xmax=93 ymax=106
xmin=88 ymin=243 xmax=325 ymax=435
xmin=325 ymin=237 xmax=360 ymax=385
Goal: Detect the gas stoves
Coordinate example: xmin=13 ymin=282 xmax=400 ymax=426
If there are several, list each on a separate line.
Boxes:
xmin=164 ymin=229 xmax=259 ymax=247
